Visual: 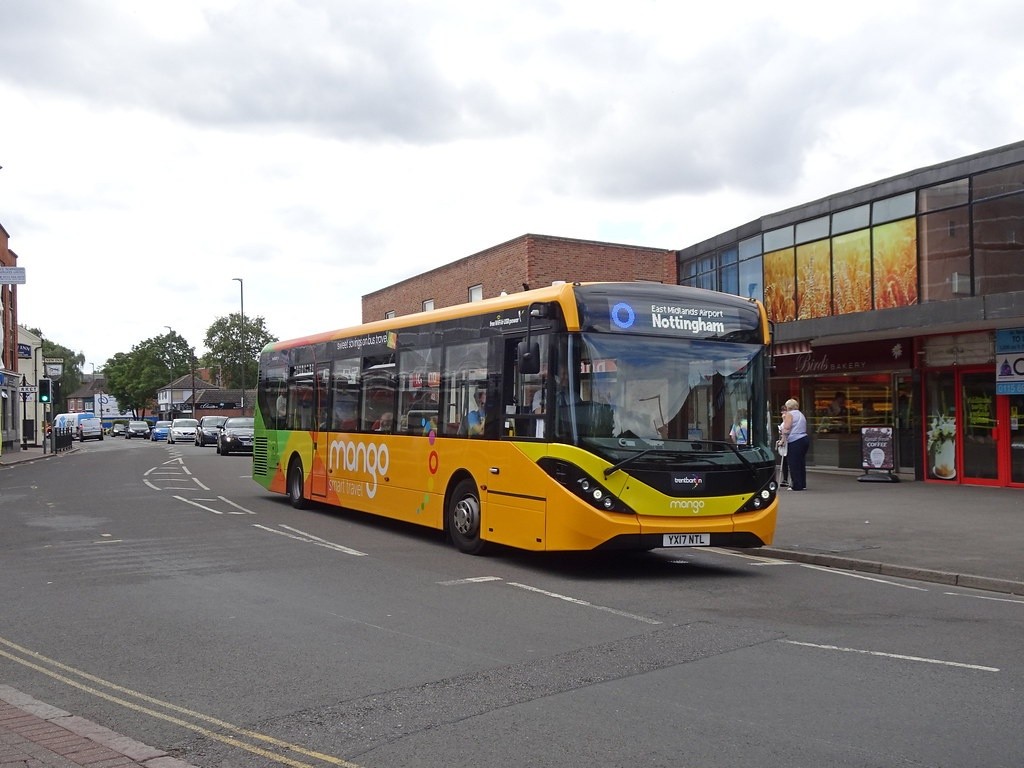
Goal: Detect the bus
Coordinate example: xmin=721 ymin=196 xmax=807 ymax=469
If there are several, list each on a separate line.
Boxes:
xmin=252 ymin=279 xmax=779 ymax=556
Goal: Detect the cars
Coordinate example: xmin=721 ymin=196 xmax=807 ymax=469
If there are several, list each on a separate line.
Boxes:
xmin=194 ymin=416 xmax=229 ymax=447
xmin=166 ymin=418 xmax=200 ymax=444
xmin=150 ymin=421 xmax=172 ymax=442
xmin=216 ymin=416 xmax=255 ymax=456
xmin=109 ymin=424 xmax=126 ymax=438
xmin=125 ymin=421 xmax=151 ymax=440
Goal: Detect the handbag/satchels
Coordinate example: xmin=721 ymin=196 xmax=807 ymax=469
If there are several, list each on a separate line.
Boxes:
xmin=777 ymin=440 xmax=788 ymax=456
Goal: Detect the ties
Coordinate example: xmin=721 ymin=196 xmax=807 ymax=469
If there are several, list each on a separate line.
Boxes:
xmin=561 ymin=391 xmax=571 ymax=435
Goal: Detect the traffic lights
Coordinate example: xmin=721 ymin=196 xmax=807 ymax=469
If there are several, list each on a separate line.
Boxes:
xmin=38 ymin=379 xmax=52 ymax=404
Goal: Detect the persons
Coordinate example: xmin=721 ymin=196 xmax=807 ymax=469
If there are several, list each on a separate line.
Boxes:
xmin=276 ymin=385 xmax=286 ymax=417
xmin=831 ymin=392 xmax=847 ymax=417
xmin=531 ymin=362 xmax=583 ymax=437
xmin=862 ymin=400 xmax=879 ymax=424
xmin=729 ymin=407 xmax=752 ymax=445
xmin=777 ymin=398 xmax=809 ymax=491
xmin=374 ymin=412 xmax=395 ymax=431
xmin=467 ymin=386 xmax=487 ymax=438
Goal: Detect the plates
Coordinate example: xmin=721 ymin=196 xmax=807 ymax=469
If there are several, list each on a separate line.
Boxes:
xmin=932 ymin=466 xmax=956 ymax=479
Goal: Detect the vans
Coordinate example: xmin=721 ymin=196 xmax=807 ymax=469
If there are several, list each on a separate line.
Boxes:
xmin=78 ymin=418 xmax=104 ymax=441
xmin=53 ymin=413 xmax=95 ymax=435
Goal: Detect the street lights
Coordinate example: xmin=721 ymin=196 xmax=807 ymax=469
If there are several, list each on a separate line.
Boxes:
xmin=183 ymin=347 xmax=195 ymax=419
xmin=164 ymin=325 xmax=173 ymax=421
xmin=232 ymin=277 xmax=245 ymax=416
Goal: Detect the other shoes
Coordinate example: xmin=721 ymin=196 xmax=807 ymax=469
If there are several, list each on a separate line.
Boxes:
xmin=787 ymin=485 xmax=807 ymax=490
